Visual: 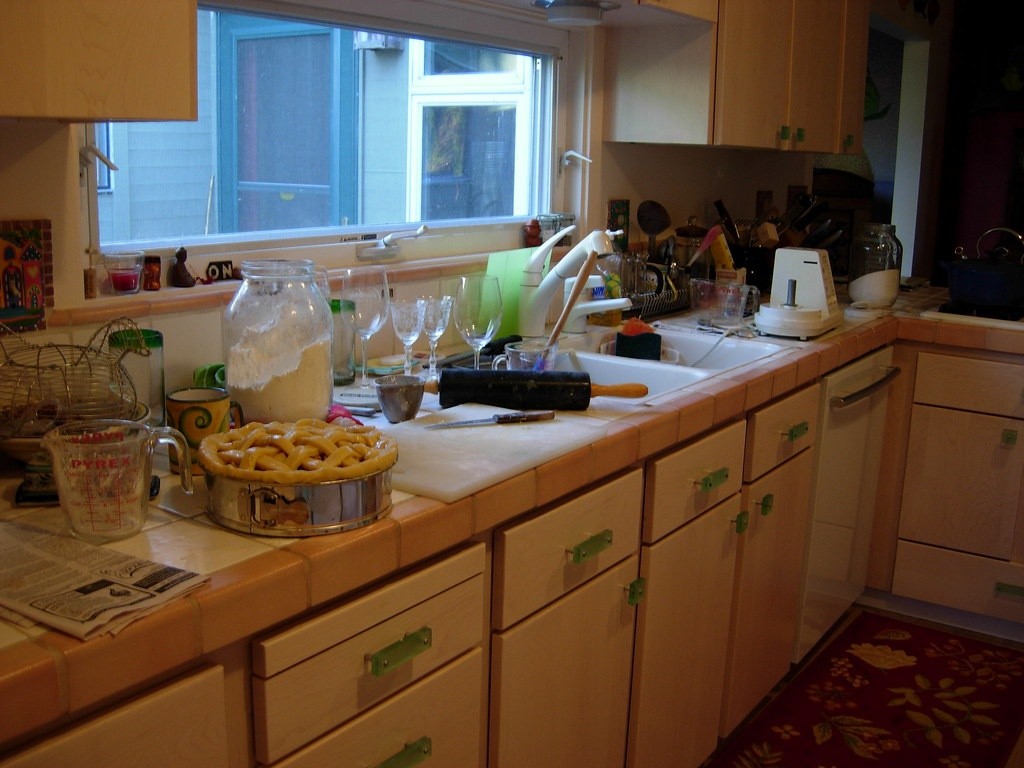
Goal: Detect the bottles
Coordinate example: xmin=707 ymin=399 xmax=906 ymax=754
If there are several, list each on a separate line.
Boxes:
xmin=847 ymin=222 xmax=903 ymax=306
xmin=222 ymin=260 xmax=334 ymax=424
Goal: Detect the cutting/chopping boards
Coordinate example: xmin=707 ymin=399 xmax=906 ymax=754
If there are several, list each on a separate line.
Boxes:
xmin=375 ymin=401 xmax=606 ymax=503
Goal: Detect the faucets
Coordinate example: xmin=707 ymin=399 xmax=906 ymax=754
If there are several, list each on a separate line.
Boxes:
xmin=516 ymin=224 xmax=634 ymax=338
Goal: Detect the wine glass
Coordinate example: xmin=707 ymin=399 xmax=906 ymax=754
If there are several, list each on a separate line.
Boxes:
xmin=421 ymin=296 xmax=451 ymax=380
xmin=340 ymin=266 xmax=391 ymax=389
xmin=389 ymin=299 xmax=427 ymax=377
xmin=453 ymin=273 xmax=502 ymax=370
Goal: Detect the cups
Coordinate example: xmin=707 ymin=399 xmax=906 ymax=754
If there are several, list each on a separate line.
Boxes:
xmin=103 ymin=250 xmax=144 ymax=294
xmin=325 ymin=297 xmax=357 ymax=385
xmin=492 ymin=339 xmax=558 ymax=372
xmin=675 ymin=226 xmax=712 ymax=279
xmin=108 ymin=329 xmax=166 ymax=427
xmin=167 ymin=387 xmax=244 ymax=476
xmin=39 ymin=422 xmax=195 ymax=541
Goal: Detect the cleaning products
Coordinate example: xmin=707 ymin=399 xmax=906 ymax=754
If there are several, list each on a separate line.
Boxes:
xmin=585 ymin=228 xmax=626 ymax=328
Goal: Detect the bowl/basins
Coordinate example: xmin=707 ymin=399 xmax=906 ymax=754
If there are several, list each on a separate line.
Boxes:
xmin=689 ymin=277 xmax=750 ymax=325
xmin=372 ymin=376 xmax=426 ymax=422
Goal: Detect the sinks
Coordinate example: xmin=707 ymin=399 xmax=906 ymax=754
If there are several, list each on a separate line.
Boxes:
xmin=492 ymin=345 xmax=709 ymax=410
xmin=564 ymin=322 xmax=790 ymax=375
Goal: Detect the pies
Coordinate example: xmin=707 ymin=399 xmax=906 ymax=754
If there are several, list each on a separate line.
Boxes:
xmin=198 ymin=418 xmax=399 ymax=484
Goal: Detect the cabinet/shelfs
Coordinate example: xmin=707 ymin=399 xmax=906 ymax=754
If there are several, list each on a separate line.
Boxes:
xmin=0 ymin=1 xmax=200 ymax=123
xmin=603 ymin=0 xmax=871 ymax=156
xmin=1 ymin=348 xmax=819 ymax=768
xmin=871 ymin=338 xmax=1024 ymax=623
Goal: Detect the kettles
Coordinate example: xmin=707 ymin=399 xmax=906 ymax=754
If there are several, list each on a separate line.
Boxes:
xmin=951 ymin=225 xmax=1024 ymax=318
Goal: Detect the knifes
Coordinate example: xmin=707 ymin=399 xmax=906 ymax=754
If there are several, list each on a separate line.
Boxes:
xmin=424 ymin=410 xmax=556 ymax=429
xmin=786 ymin=192 xmax=843 ymax=250
